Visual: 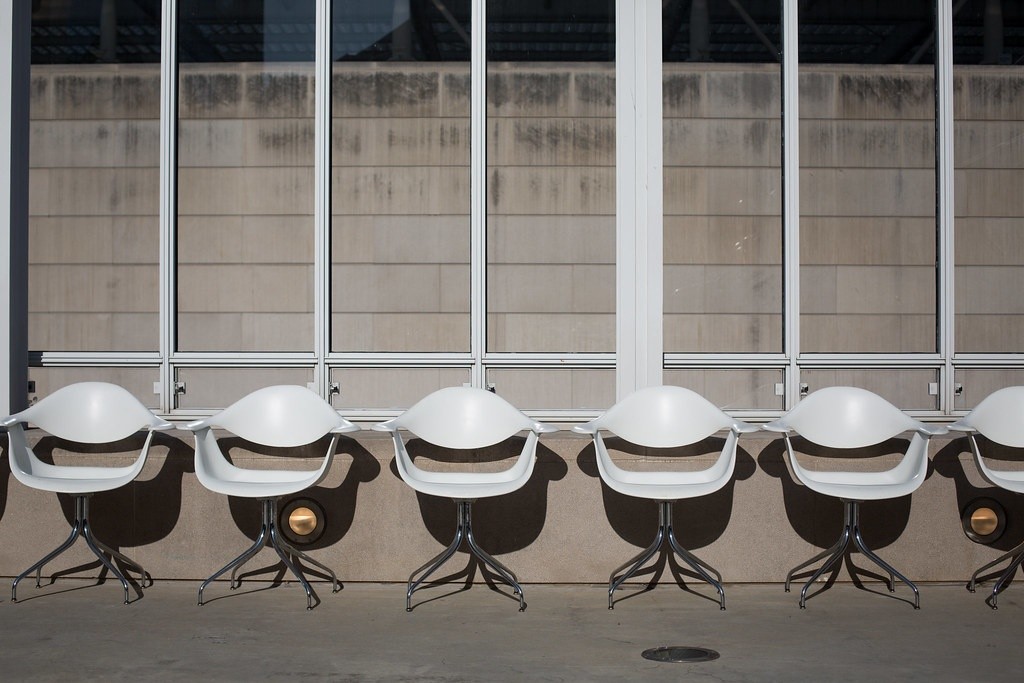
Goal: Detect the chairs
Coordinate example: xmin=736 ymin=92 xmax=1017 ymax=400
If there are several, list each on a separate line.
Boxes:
xmin=761 ymin=386 xmax=950 ymax=610
xmin=572 ymin=386 xmax=761 ymax=611
xmin=2 ymin=382 xmax=176 ymax=605
xmin=176 ymin=384 xmax=361 ymax=611
xmin=946 ymin=386 xmax=1024 ymax=609
xmin=370 ymin=386 xmax=560 ymax=612
